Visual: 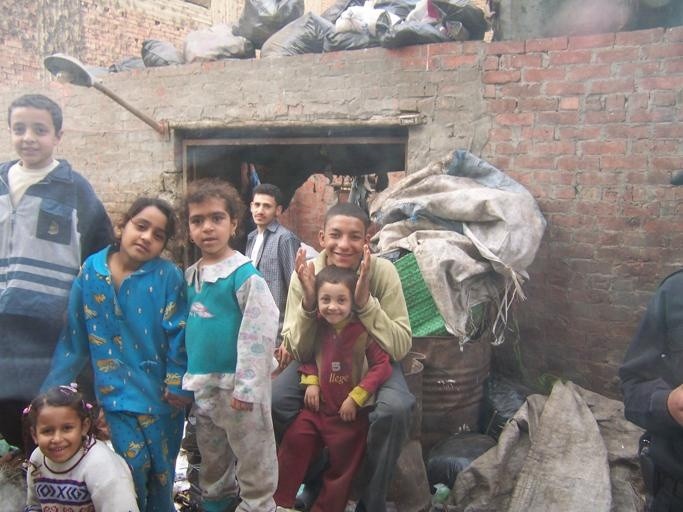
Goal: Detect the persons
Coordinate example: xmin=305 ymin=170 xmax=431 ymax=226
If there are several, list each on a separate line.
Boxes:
xmin=158 ymin=177 xmax=281 ymax=511
xmin=243 ymin=182 xmax=300 ymax=380
xmin=19 ymin=383 xmax=140 ymax=512
xmin=272 ymin=264 xmax=392 ymax=512
xmin=0 ymin=92 xmax=119 ymax=477
xmin=618 ymin=269 xmax=681 ymax=512
xmin=36 ymin=195 xmax=193 ymax=512
xmin=269 ymin=202 xmax=416 ymax=512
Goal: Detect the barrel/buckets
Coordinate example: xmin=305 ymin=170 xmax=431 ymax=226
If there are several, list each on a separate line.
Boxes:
xmin=410 ymin=325 xmax=491 ymax=458
xmin=348 ymin=357 xmax=431 ymax=512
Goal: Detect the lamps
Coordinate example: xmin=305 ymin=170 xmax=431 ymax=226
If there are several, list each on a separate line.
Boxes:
xmin=42 ymin=53 xmax=171 ymax=143
